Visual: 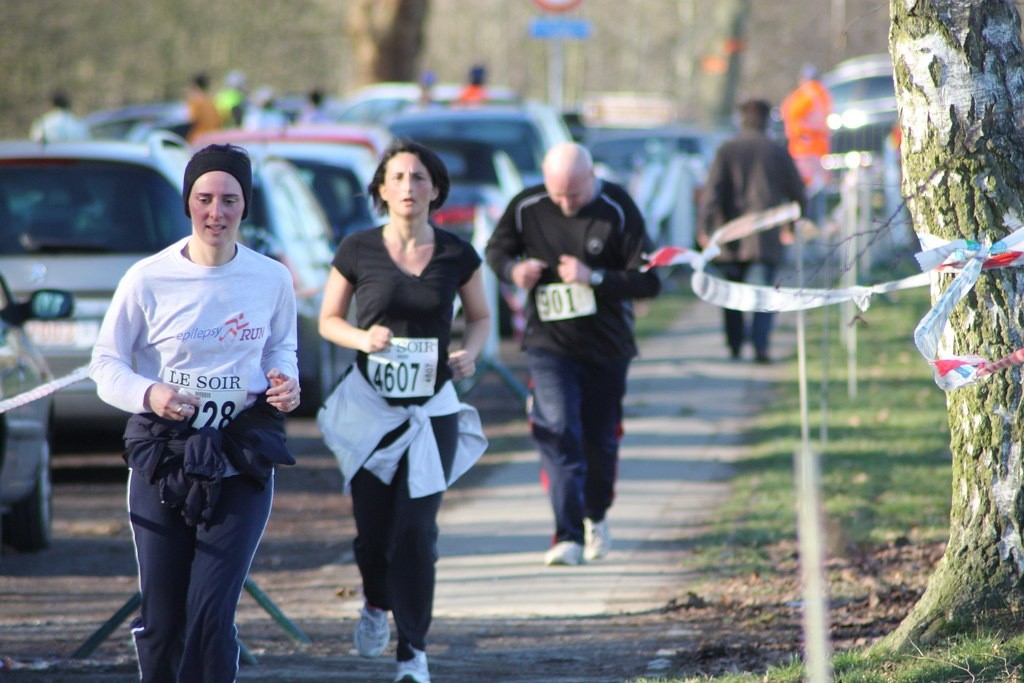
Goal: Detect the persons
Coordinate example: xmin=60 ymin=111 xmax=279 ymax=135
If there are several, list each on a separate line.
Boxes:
xmin=27 ymin=67 xmax=661 ymax=682
xmin=695 ymin=64 xmax=833 ymax=363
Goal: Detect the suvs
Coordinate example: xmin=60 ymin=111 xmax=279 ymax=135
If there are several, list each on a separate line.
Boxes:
xmin=0 ymin=130 xmax=248 ymax=465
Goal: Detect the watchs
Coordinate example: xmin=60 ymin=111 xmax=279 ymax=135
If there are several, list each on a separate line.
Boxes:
xmin=589 ymin=269 xmax=606 ymax=286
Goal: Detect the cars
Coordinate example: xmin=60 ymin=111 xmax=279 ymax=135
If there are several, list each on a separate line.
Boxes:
xmin=85 ymin=78 xmax=735 ymax=416
xmin=0 ymin=269 xmax=72 ymax=553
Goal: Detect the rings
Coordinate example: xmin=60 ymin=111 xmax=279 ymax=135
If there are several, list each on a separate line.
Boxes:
xmin=292 ymin=401 xmax=295 ymax=406
xmin=177 ymin=408 xmax=183 ymax=415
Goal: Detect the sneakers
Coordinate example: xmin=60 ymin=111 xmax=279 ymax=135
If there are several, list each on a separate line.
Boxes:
xmin=583 ymin=517 xmax=611 ymax=559
xmin=394 ymin=643 xmax=430 ymax=683
xmin=354 ymin=601 xmax=389 ymax=658
xmin=546 ymin=540 xmax=581 ymax=565
